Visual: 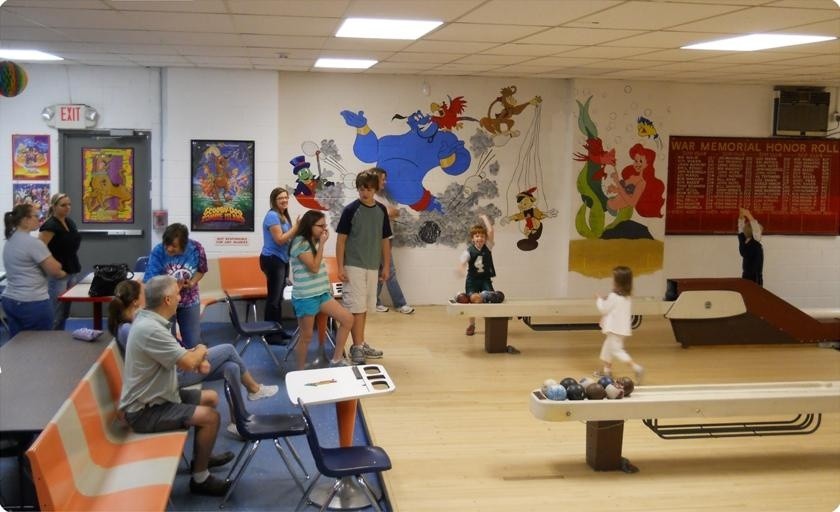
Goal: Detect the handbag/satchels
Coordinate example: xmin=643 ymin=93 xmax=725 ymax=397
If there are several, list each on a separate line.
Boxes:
xmin=71 ymin=326 xmax=105 ymax=341
xmin=88 ymin=262 xmax=133 ymax=297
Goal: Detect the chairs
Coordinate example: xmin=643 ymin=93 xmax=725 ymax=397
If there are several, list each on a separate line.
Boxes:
xmin=283 ymin=282 xmax=347 ymax=364
xmin=297 ymin=400 xmax=393 ymax=512
xmin=220 ymin=251 xmax=349 ymax=331
xmin=23 ymin=254 xmax=229 ymax=512
xmin=219 ymin=369 xmax=310 ymax=507
xmin=222 ymin=289 xmax=284 ymax=372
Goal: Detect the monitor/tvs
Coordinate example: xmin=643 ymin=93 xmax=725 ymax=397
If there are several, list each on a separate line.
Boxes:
xmin=776 ymin=103 xmax=829 ymax=137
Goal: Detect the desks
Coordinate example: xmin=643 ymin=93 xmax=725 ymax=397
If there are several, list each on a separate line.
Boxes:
xmin=285 ymin=363 xmax=396 ymax=510
xmin=55 ymin=272 xmax=146 ymax=301
xmin=0 ymin=329 xmax=118 ymax=430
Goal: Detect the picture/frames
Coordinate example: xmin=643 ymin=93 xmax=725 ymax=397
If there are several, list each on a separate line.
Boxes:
xmin=190 ymin=139 xmax=256 ymax=232
xmin=12 ymin=183 xmax=50 ymax=229
xmin=80 ymin=146 xmax=136 ymax=223
xmin=12 ymin=134 xmax=51 ymax=181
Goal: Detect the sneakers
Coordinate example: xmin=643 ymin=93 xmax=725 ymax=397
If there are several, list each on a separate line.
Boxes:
xmin=192 ymin=451 xmax=234 ymax=468
xmin=362 ymin=342 xmax=384 ymax=360
xmin=376 ymin=305 xmax=389 ymax=312
xmin=466 ymin=325 xmax=475 ymax=335
xmin=595 ymin=369 xmax=613 ymax=378
xmin=396 ymin=305 xmax=415 ymax=314
xmin=349 ymin=345 xmax=366 ymax=365
xmin=634 ymin=366 xmax=643 ymax=387
xmin=329 ymin=357 xmax=354 ymax=366
xmin=191 ymin=476 xmax=230 ymax=496
xmin=247 ymin=383 xmax=279 ymax=400
xmin=227 ymin=421 xmax=242 ymax=442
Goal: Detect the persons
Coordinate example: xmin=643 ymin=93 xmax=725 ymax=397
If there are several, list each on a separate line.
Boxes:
xmin=594 ymin=267 xmax=644 ymax=386
xmin=38 ymin=193 xmax=81 ymax=331
xmin=260 ymin=188 xmax=302 ymax=346
xmin=142 ymin=223 xmax=208 ymax=350
xmin=287 ymin=210 xmax=354 ymax=370
xmin=1 ymin=204 xmax=67 ymax=340
xmin=288 ymin=153 xmax=335 ymax=213
xmin=738 ymin=208 xmax=763 ymax=289
xmin=335 ymin=170 xmax=395 ymax=365
xmin=118 ymin=274 xmax=235 ymax=498
xmin=607 ymin=141 xmax=664 ymax=228
xmin=369 ymin=168 xmax=415 ymax=314
xmin=458 ymin=211 xmax=497 ymax=336
xmin=108 ymin=281 xmax=279 ymax=438
xmin=500 ymin=187 xmax=559 ymax=253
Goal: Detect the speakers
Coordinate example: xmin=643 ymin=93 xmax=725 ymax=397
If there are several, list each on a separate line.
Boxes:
xmin=780 ymin=90 xmax=800 ymax=103
xmin=809 ymin=92 xmax=830 ymax=103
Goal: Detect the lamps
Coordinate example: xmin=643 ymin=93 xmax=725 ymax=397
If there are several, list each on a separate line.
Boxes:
xmin=41 ymin=104 xmax=102 ymax=131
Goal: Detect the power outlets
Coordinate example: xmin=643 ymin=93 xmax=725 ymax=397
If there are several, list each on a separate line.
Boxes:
xmin=837 ymin=112 xmax=840 ymax=123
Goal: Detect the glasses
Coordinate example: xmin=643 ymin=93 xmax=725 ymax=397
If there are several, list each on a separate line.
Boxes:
xmin=276 ymin=196 xmax=290 ymax=201
xmin=312 ymin=223 xmax=326 ymax=230
